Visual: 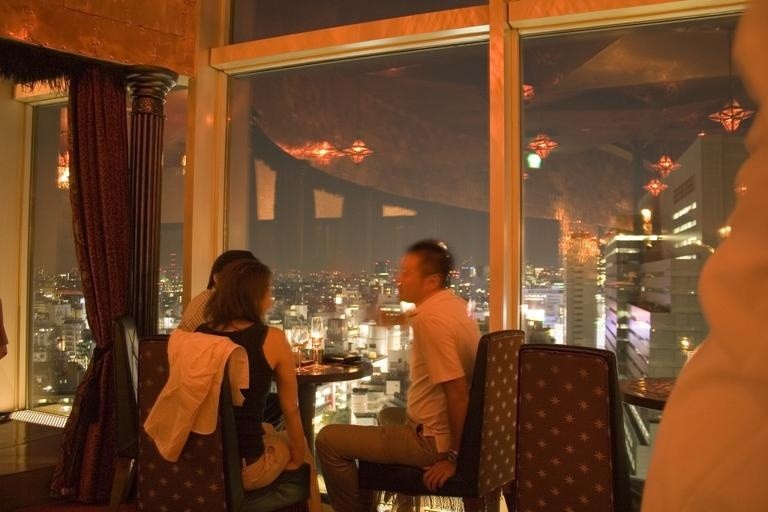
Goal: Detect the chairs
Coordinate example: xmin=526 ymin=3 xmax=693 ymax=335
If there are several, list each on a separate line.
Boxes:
xmin=513 ymin=343 xmax=645 ymax=512
xmin=136 ymin=334 xmax=311 ymax=512
xmin=355 ymin=329 xmax=526 ymax=512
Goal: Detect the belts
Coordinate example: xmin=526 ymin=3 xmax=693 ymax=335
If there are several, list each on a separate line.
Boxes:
xmin=239 ymin=455 xmax=261 ymax=469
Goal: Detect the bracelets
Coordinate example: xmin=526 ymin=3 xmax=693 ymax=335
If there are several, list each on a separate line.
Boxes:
xmin=448 ymin=448 xmax=458 ymax=464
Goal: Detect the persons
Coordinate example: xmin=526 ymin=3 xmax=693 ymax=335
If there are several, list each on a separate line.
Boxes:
xmin=192 ymin=257 xmax=324 ymax=512
xmin=314 ymin=237 xmax=482 ymax=511
xmin=177 ymin=249 xmax=256 ymax=333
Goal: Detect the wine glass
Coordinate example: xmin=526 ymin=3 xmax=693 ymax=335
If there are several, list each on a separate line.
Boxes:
xmin=291 ymin=324 xmax=309 ymax=373
xmin=310 ymin=316 xmax=325 ymax=371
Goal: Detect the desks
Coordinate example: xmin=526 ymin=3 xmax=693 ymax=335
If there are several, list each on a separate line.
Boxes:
xmin=294 ymin=360 xmax=373 ymax=458
xmin=618 ymin=376 xmax=679 ymax=410
